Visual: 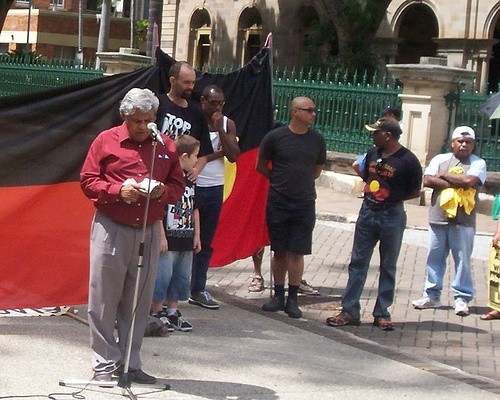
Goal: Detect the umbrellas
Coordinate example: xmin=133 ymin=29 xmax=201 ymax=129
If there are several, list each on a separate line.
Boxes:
xmin=478 ymin=90 xmax=500 ymax=120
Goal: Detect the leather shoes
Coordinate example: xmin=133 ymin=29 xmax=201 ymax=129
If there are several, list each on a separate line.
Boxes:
xmin=94 ymin=370 xmax=114 ymax=387
xmin=284 ymin=299 xmax=302 ymax=318
xmin=262 ymin=298 xmax=284 ymax=311
xmin=119 ymin=369 xmax=157 ymax=384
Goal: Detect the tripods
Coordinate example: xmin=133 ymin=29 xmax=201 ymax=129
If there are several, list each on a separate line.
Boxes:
xmin=59 ymin=140 xmax=170 ymax=400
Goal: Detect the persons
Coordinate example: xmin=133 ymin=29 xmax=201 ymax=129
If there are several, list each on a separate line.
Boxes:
xmin=255 ymin=96 xmax=326 ymax=318
xmin=150 ymin=61 xmax=215 ymax=184
xmin=326 ymin=118 xmax=422 ymax=331
xmin=188 ymin=84 xmax=240 ymax=308
xmin=80 ymin=87 xmax=187 ymax=387
xmin=480 ymin=192 xmax=500 ymax=320
xmin=412 ymin=125 xmax=487 ymax=316
xmin=248 ymin=121 xmax=320 ymax=296
xmin=151 ymin=134 xmax=202 ymax=332
xmin=352 ymin=105 xmax=403 ymax=198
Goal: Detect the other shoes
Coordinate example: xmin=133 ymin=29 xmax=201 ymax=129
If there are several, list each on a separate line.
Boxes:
xmin=480 ymin=310 xmax=500 ymax=319
xmin=248 ymin=275 xmax=264 ymax=292
xmin=297 ymin=284 xmax=313 ymax=292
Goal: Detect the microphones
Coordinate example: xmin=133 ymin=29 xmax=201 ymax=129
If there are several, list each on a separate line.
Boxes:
xmin=147 ymin=122 xmax=165 ymax=146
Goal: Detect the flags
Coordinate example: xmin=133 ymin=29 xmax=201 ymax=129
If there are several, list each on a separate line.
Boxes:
xmin=0 ymin=64 xmax=161 ymax=309
xmin=154 ymin=44 xmax=270 ymax=268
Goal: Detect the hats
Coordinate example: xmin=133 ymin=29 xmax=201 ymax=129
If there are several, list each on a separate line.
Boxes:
xmin=452 ymin=126 xmax=475 ymax=140
xmin=381 ymin=107 xmax=399 ymax=115
xmin=364 ymin=118 xmax=402 ymax=135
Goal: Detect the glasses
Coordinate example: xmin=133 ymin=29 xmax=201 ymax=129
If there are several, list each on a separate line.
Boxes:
xmin=205 ymin=97 xmax=225 ymax=106
xmin=296 ymin=107 xmax=316 ymax=113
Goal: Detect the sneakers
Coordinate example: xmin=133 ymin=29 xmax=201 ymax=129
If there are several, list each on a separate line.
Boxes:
xmin=374 ymin=316 xmax=393 ymax=329
xmin=165 ymin=309 xmax=192 ymax=331
xmin=412 ymin=297 xmax=441 ymax=308
xmin=326 ymin=311 xmax=360 ymax=325
xmin=188 ymin=291 xmax=219 ymax=307
xmin=152 ymin=311 xmax=174 ymax=331
xmin=455 ymin=297 xmax=469 ymax=315
